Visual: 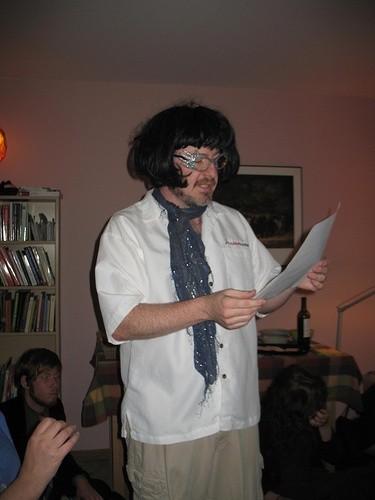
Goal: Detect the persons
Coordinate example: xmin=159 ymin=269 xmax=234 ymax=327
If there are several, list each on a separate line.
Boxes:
xmin=0 ymin=347 xmax=129 ymax=500
xmin=95 ymin=100 xmax=329 ymax=500
xmin=0 ymin=412 xmax=79 ymax=499
xmin=261 ymin=363 xmax=375 ymax=500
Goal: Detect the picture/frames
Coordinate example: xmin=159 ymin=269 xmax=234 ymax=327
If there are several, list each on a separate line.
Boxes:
xmin=212 ymin=164 xmax=303 ymax=266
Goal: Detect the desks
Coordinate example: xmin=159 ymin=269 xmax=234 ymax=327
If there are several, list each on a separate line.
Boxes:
xmin=82 ymin=330 xmax=363 ymax=500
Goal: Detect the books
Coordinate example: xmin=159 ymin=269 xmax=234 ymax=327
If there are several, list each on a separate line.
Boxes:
xmin=0 ymin=357 xmax=23 ymax=405
xmin=0 ymin=246 xmax=56 ymax=287
xmin=0 ymin=290 xmax=57 ymax=333
xmin=0 ymin=201 xmax=57 ymax=242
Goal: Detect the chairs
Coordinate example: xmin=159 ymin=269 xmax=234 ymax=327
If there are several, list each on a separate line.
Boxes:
xmin=0 ymin=195 xmax=62 ymax=403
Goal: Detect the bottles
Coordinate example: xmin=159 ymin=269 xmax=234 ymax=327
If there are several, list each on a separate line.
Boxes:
xmin=295 ymin=297 xmax=312 ymax=350
